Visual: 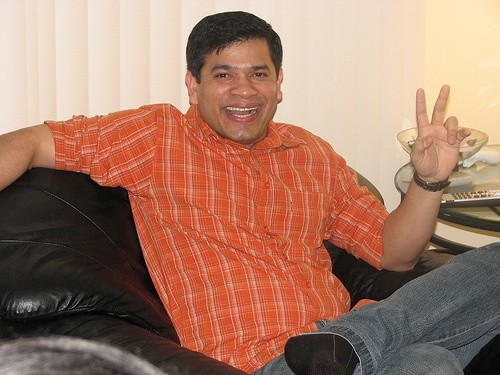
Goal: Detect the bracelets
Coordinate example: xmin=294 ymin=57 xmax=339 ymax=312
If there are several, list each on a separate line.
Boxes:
xmin=413 ymin=171 xmax=452 ymax=192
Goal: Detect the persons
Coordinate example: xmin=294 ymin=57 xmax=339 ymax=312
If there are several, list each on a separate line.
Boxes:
xmin=0 ymin=12 xmax=500 ymax=375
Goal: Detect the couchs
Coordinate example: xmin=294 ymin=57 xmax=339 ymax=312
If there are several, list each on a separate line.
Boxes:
xmin=0 ymin=167 xmax=500 ymax=374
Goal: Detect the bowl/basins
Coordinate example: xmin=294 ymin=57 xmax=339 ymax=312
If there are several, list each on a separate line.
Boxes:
xmin=396 ymin=126 xmax=489 ymax=172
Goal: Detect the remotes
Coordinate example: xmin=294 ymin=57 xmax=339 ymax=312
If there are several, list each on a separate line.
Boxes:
xmin=440 ymin=190 xmax=500 ymax=208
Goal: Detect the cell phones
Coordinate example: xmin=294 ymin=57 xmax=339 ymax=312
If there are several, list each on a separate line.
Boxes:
xmin=448 ymin=172 xmax=473 ymax=187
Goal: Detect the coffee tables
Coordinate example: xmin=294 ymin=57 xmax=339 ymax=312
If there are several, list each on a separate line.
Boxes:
xmin=394 ymin=145 xmax=500 ymax=254
